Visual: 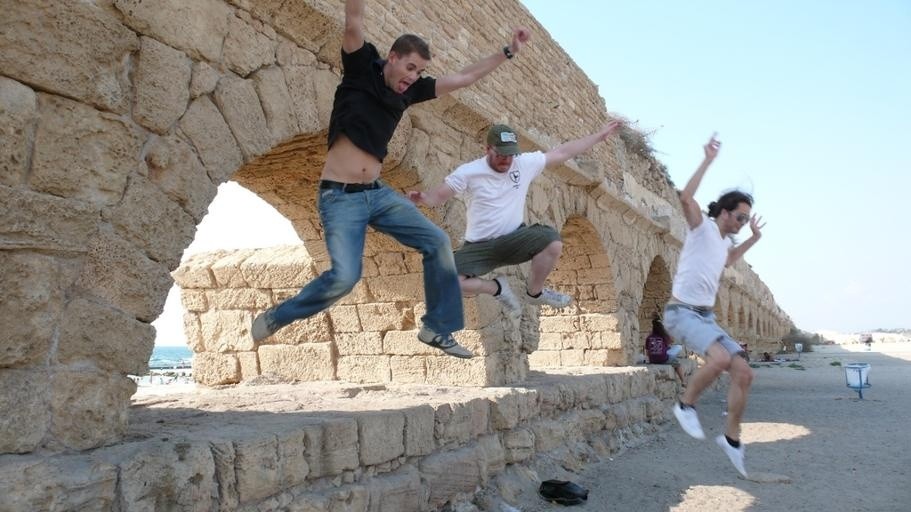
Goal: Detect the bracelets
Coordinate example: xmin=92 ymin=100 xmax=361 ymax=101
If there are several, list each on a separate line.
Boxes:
xmin=503 ymin=44 xmax=514 ymax=60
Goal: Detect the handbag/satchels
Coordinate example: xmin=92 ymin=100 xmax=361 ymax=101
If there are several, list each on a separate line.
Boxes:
xmin=539 ymin=479 xmax=588 ymax=505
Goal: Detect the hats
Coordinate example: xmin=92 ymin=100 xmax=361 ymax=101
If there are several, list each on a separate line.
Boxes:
xmin=488 ymin=125 xmax=521 ymax=156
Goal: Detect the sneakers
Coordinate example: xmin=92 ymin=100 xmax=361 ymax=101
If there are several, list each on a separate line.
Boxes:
xmin=526 ymin=287 xmax=572 ymax=309
xmin=417 ymin=323 xmax=473 ymax=359
xmin=716 ymin=434 xmax=747 ymax=478
xmin=494 ymin=276 xmax=520 ymax=312
xmin=673 ymin=402 xmax=705 ymax=440
xmin=251 ymin=307 xmax=283 ymax=340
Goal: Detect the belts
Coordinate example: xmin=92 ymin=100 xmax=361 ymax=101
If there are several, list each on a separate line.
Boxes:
xmin=319 ymin=180 xmax=382 ymax=193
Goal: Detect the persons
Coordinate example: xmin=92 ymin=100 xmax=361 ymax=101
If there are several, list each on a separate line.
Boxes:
xmin=407 ymin=119 xmax=624 ymax=318
xmin=250 ymin=1 xmax=530 ymax=359
xmin=645 ymin=319 xmax=687 ymax=388
xmin=660 ymin=133 xmax=767 ymax=480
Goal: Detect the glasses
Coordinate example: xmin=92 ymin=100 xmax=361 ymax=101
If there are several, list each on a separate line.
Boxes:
xmin=489 ymin=146 xmax=517 ymax=158
xmin=730 ymin=213 xmax=750 ymax=223
